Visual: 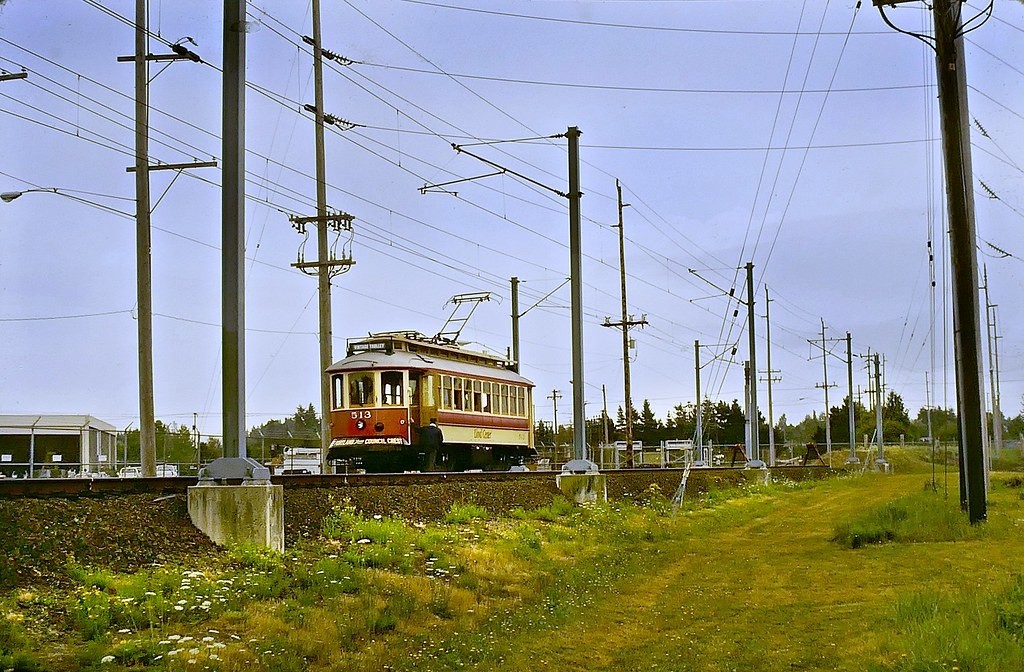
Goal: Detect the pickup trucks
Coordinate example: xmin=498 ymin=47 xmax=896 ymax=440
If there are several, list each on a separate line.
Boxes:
xmin=118 ymin=465 xmax=178 ymax=476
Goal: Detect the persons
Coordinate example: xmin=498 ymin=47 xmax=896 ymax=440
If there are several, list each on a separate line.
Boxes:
xmin=411 ymin=417 xmax=444 ymax=472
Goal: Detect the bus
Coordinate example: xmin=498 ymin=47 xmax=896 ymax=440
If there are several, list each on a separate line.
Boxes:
xmin=327 ymin=331 xmax=537 ymax=472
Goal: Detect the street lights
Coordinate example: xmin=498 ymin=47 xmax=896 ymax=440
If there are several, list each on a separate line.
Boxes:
xmin=1 ymin=189 xmax=157 ymax=477
